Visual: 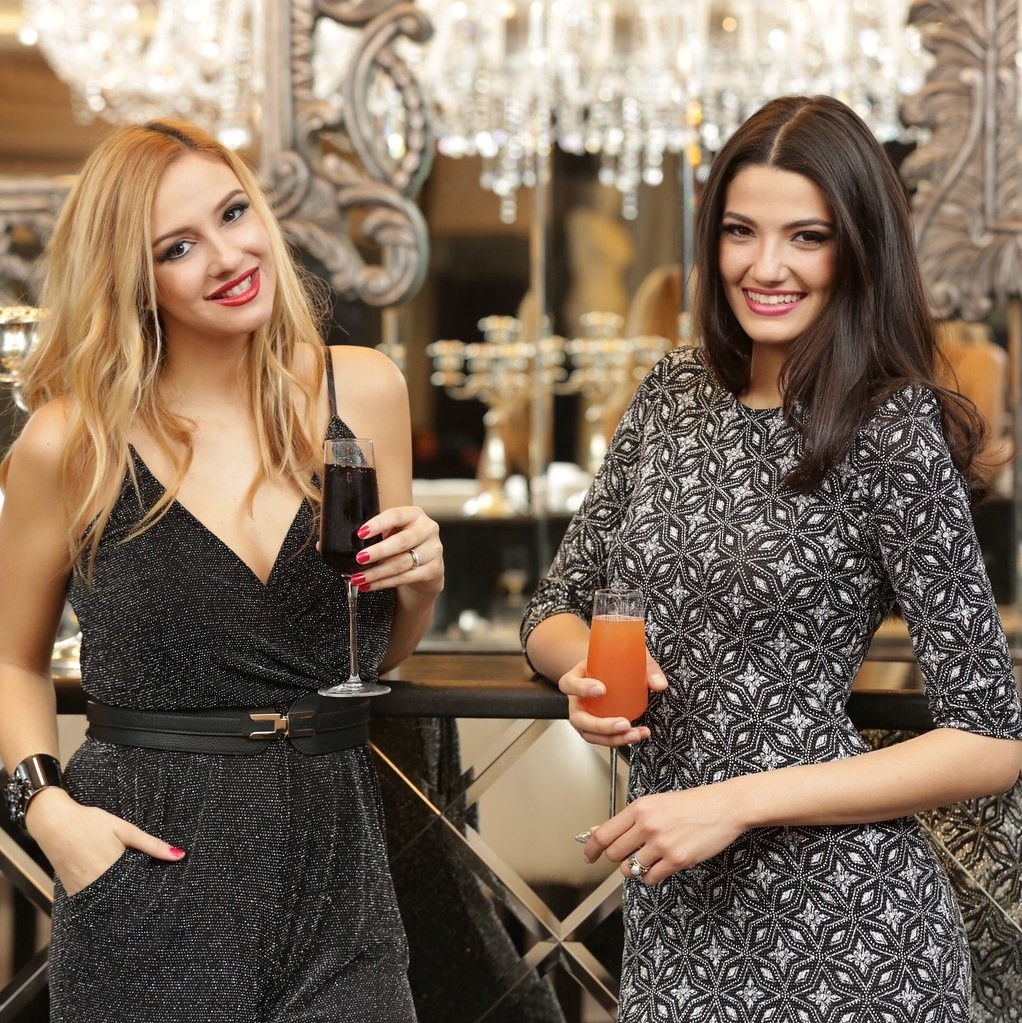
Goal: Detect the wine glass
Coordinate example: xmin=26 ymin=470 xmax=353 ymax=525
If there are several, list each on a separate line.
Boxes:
xmin=318 ymin=436 xmax=393 ymax=699
xmin=572 ymin=589 xmax=652 ymax=845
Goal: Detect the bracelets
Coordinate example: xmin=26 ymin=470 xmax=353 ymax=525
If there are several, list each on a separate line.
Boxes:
xmin=5 ymin=753 xmax=64 ymax=827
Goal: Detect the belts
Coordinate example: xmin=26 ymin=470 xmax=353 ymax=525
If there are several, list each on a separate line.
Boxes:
xmin=82 ymin=697 xmax=378 ymax=756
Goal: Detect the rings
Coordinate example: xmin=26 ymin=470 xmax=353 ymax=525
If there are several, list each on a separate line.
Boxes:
xmin=628 ymin=852 xmax=653 ymax=878
xmin=407 ymin=549 xmax=419 ymax=570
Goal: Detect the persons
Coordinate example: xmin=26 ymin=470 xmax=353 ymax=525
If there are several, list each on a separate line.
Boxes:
xmin=519 ymin=94 xmax=1022 ymax=1023
xmin=0 ymin=117 xmax=450 ymax=1022
